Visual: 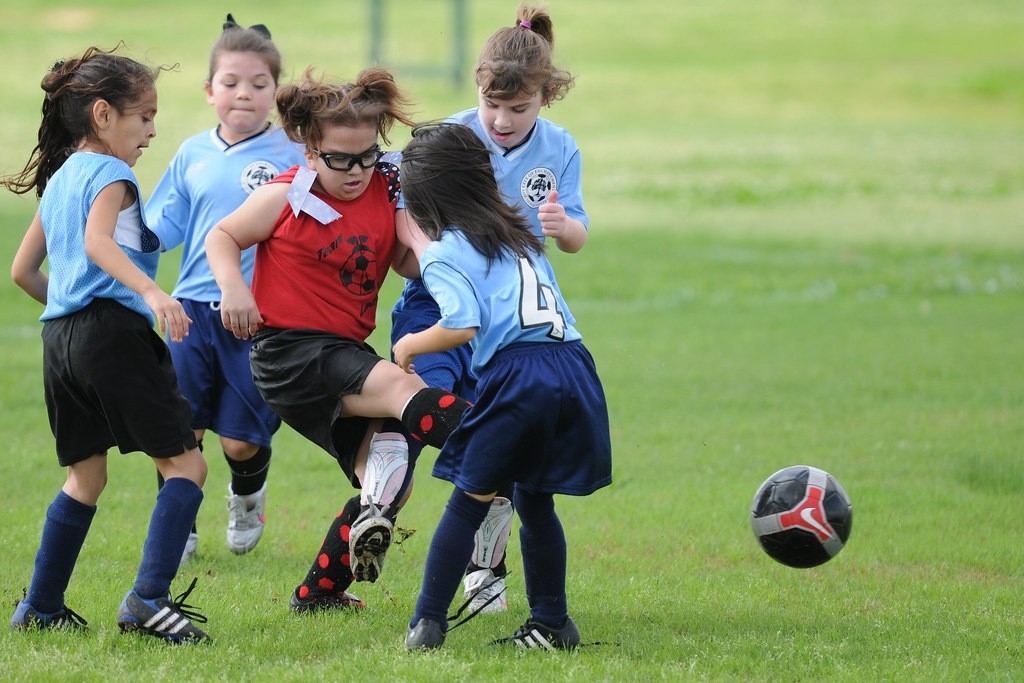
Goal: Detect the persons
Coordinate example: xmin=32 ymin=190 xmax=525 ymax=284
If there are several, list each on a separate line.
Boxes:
xmin=205 ymin=67 xmax=475 ymax=609
xmin=349 ymin=3 xmax=589 ymax=615
xmin=392 ymin=122 xmax=612 ymax=655
xmin=144 ymin=14 xmax=307 ymax=560
xmin=0 ymin=41 xmax=216 ymax=646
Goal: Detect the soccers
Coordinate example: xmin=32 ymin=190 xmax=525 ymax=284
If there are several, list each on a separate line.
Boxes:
xmin=748 ymin=463 xmax=855 ymax=570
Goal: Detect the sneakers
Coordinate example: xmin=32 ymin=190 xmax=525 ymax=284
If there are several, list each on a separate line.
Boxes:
xmin=484 ymin=619 xmax=580 ymax=662
xmin=405 ymin=619 xmax=447 ymax=658
xmin=180 ymin=529 xmax=198 ymax=563
xmin=224 ymin=480 xmax=267 ymax=555
xmin=349 ymin=495 xmax=394 ymax=584
xmin=9 ymin=601 xmax=94 ymax=637
xmin=116 ymin=578 xmax=214 ymax=647
xmin=465 ymin=570 xmax=507 ymax=618
xmin=290 ymin=587 xmax=365 ymax=617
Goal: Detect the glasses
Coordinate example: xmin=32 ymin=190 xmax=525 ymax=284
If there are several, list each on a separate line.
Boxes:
xmin=313 ymin=149 xmax=386 ymax=170
